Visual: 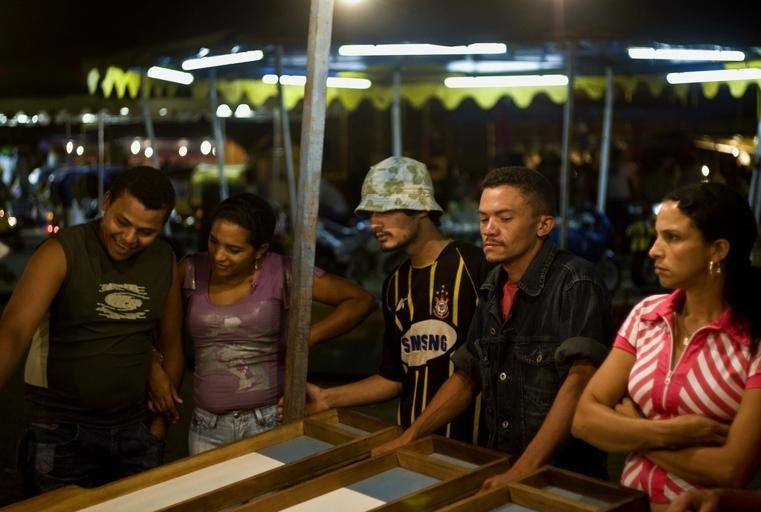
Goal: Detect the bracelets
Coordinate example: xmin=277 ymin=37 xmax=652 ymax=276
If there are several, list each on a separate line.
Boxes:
xmin=681 ymin=335 xmax=690 ymax=346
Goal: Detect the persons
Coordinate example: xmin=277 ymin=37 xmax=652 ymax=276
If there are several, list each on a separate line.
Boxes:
xmin=550 ymin=201 xmax=660 ymax=288
xmin=568 ymin=179 xmax=761 ymax=504
xmin=275 ymin=154 xmax=495 ymax=448
xmin=145 ymin=191 xmax=375 ymax=457
xmin=1 ymin=166 xmax=184 ymax=489
xmin=366 ymin=162 xmax=614 ymax=495
xmin=666 ymin=490 xmax=761 ymax=512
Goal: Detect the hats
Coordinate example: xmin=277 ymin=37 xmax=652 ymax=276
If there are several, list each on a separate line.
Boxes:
xmin=354 ymin=156 xmax=445 ymax=218
